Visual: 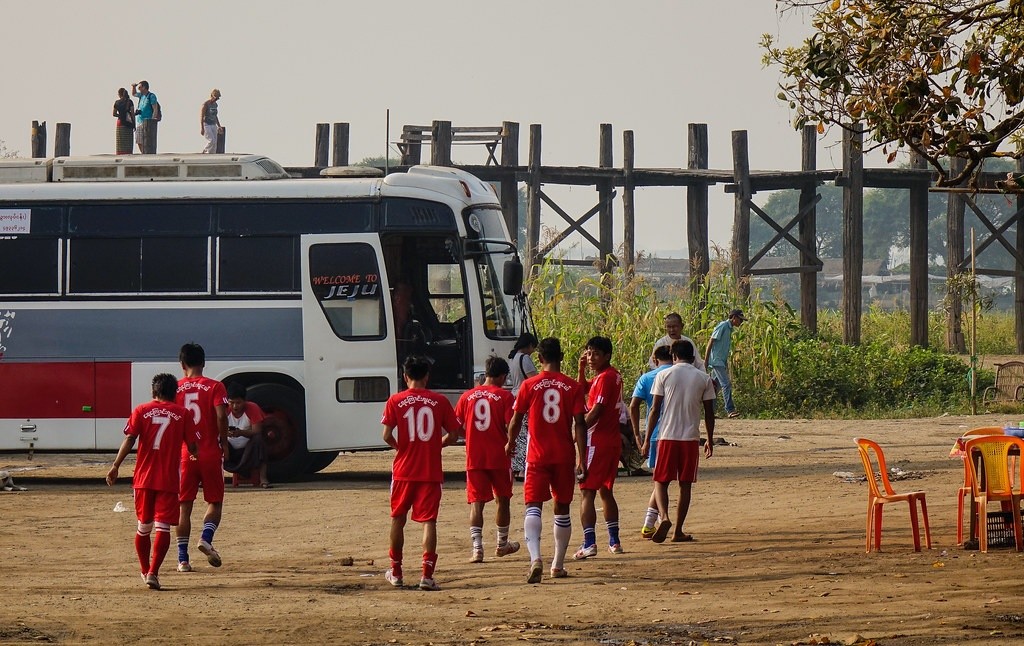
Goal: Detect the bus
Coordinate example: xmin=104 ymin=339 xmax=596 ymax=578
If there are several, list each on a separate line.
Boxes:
xmin=0 ymin=152 xmax=538 ymax=482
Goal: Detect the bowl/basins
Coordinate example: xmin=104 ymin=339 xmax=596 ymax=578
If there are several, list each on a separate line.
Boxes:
xmin=1003 ymin=428 xmax=1024 ymax=438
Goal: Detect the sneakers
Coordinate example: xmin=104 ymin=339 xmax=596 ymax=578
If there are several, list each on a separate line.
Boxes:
xmin=198 ymin=538 xmax=222 ymax=567
xmin=385 ymin=569 xmax=404 ymax=588
xmin=420 ymin=576 xmax=440 ymax=590
xmin=469 ymin=551 xmax=483 ymax=562
xmin=550 ymin=566 xmax=567 ymax=578
xmin=177 ymin=561 xmax=191 ymax=572
xmin=495 ymin=540 xmax=521 ymax=557
xmin=141 ymin=573 xmax=147 ymax=584
xmin=608 ymin=544 xmax=623 ymax=555
xmin=572 ymin=544 xmax=597 ymax=560
xmin=642 ymin=526 xmax=658 ymax=538
xmin=526 ymin=557 xmax=543 ymax=583
xmin=147 ymin=572 xmax=160 ymax=590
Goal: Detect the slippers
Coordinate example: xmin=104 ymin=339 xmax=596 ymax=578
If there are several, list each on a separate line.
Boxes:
xmin=652 ymin=520 xmax=672 ymax=543
xmin=671 ymin=535 xmax=693 ymax=542
xmin=728 ymin=412 xmax=740 ymax=418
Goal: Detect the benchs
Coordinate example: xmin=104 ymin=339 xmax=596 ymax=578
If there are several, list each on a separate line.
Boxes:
xmin=390 ymin=124 xmax=502 ymax=165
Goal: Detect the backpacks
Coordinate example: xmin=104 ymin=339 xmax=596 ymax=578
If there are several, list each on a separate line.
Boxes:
xmin=148 ymin=93 xmax=162 ymax=121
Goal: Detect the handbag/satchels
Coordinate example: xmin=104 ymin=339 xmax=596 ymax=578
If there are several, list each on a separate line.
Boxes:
xmin=123 ymin=111 xmax=134 ymax=128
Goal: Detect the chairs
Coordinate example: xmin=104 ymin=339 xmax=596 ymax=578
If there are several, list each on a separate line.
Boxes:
xmin=983 ymin=361 xmax=1024 ymax=407
xmin=413 ymin=287 xmax=457 ymax=340
xmin=853 ymin=437 xmax=930 ymax=553
xmin=964 ymin=434 xmax=1024 ymax=553
xmin=957 ymin=426 xmax=1014 ymax=547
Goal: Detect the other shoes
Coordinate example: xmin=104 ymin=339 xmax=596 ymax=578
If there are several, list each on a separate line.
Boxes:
xmin=514 ymin=472 xmax=525 ymax=481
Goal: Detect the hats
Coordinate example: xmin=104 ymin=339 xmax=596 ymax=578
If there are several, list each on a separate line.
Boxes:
xmin=729 ymin=309 xmax=748 ymax=322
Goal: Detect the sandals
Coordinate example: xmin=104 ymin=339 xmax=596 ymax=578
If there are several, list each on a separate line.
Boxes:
xmin=260 ymin=481 xmax=274 ymax=489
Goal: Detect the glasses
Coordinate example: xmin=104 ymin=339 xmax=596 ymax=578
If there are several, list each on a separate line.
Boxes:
xmin=138 ymin=87 xmax=141 ymax=88
xmin=213 ymin=96 xmax=220 ymax=101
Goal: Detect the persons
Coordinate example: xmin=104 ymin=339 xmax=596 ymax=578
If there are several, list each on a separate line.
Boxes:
xmin=454 ymin=353 xmax=520 ymax=561
xmin=106 ymin=373 xmax=198 ymax=589
xmin=223 ymin=382 xmax=273 ymax=489
xmin=381 ymin=355 xmax=459 ymax=590
xmin=200 ymin=89 xmax=223 ymax=154
xmin=131 ymin=81 xmax=157 ymax=154
xmin=175 ymin=343 xmax=230 ymax=573
xmin=703 ymin=309 xmax=749 ymax=419
xmin=112 ymin=88 xmax=137 ymax=155
xmin=508 ymin=311 xmax=707 ymax=584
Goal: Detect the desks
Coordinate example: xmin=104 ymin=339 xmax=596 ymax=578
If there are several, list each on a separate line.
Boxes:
xmin=948 ymin=436 xmax=1024 ymax=542
